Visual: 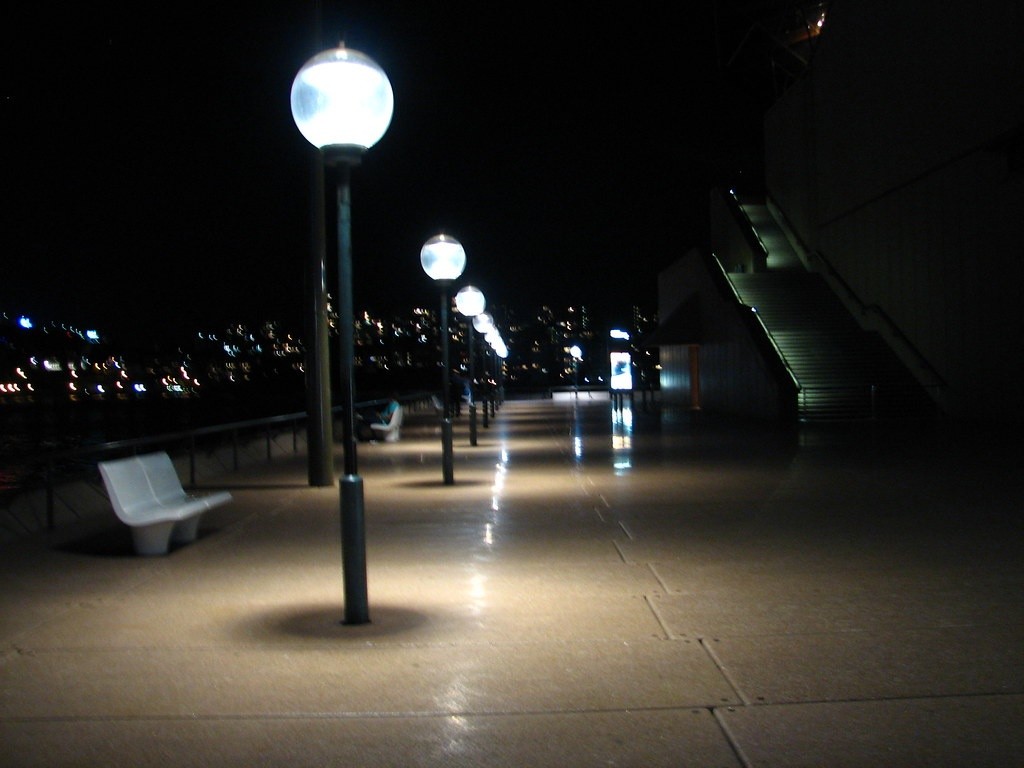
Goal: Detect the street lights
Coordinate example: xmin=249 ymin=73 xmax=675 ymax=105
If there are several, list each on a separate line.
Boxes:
xmin=473 ymin=312 xmax=508 ymax=428
xmin=290 ymin=40 xmax=394 ymax=625
xmin=421 ymin=234 xmax=467 ymax=485
xmin=456 ymin=285 xmax=486 ymax=445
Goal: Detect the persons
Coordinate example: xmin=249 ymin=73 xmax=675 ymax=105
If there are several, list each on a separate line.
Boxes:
xmin=355 ymin=395 xmax=400 ymax=443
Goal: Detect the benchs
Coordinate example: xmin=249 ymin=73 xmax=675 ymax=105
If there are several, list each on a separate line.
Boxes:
xmin=96 ymin=450 xmax=233 ymax=553
xmin=371 ymin=407 xmax=405 ymax=446
